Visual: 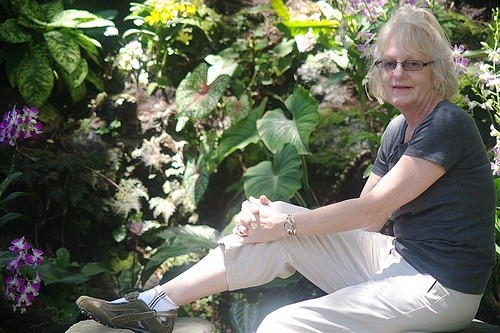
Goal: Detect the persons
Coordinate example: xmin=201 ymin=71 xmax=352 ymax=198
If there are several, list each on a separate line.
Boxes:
xmin=76 ymin=6 xmax=495 ymax=333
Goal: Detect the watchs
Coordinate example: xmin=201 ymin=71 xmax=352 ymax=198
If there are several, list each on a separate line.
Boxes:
xmin=284 ymin=213 xmax=297 ymax=235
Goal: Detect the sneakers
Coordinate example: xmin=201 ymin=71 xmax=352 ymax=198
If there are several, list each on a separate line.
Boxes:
xmin=76 ymin=291 xmax=176 ymax=333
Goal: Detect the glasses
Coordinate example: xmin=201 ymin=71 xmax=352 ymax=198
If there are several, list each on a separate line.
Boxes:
xmin=374 ymin=59 xmax=434 ymax=71
